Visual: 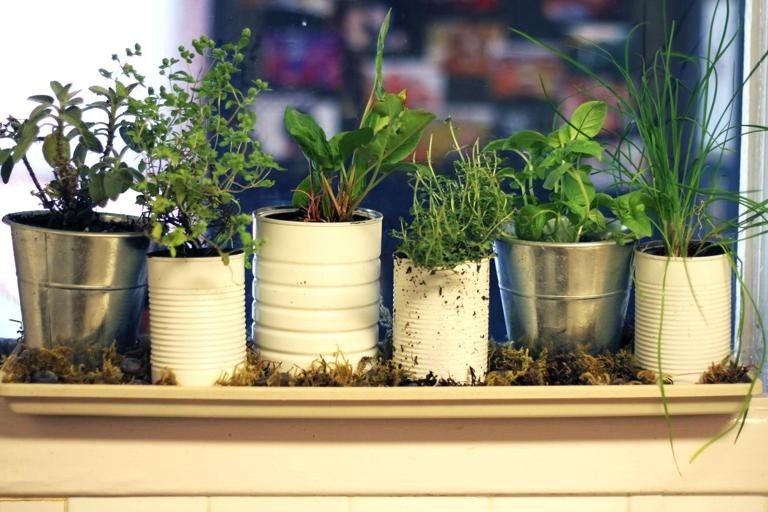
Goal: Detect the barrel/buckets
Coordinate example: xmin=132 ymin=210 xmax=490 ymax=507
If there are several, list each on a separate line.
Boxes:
xmin=1 ymin=210 xmax=152 ymax=361
xmin=490 ymin=216 xmax=634 ymax=357
